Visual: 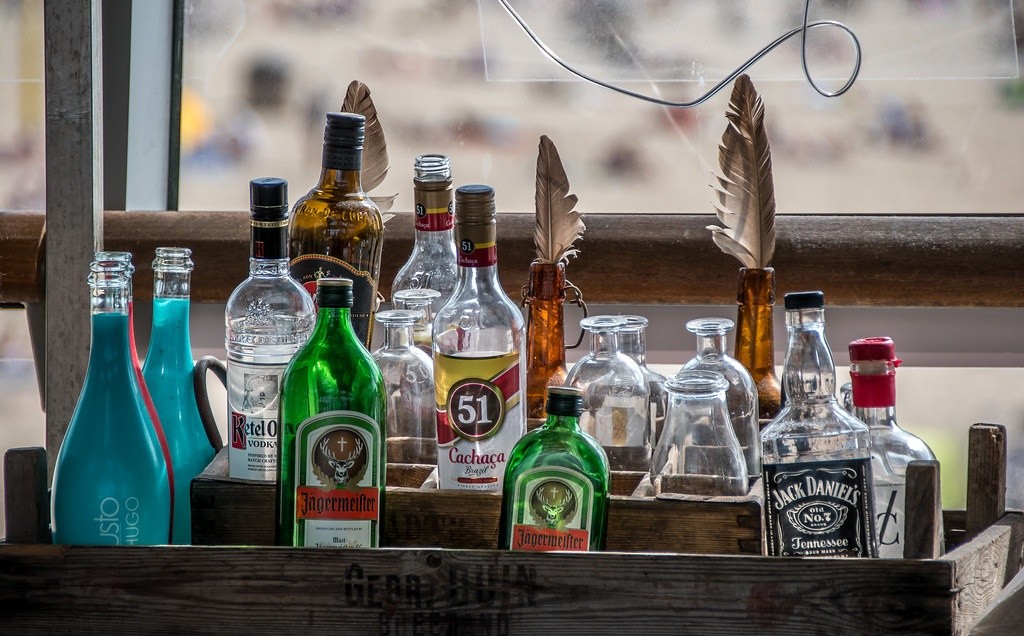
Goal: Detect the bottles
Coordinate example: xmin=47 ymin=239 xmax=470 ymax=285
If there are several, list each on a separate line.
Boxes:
xmin=94 ymin=250 xmax=175 ymax=545
xmin=496 ymin=386 xmax=612 ymax=550
xmin=586 ymin=316 xmax=669 ymax=419
xmin=649 ymin=370 xmax=749 ymax=495
xmin=391 ymin=153 xmax=460 ymax=311
xmin=276 ymin=276 xmax=385 ymax=547
xmin=137 ymin=246 xmax=216 ymax=546
xmin=522 ymin=259 xmax=568 ymax=431
xmin=371 ymin=310 xmax=434 ymax=465
xmin=729 ymin=268 xmax=782 ymax=418
xmin=48 ymin=261 xmax=171 ymax=546
xmin=836 ymin=336 xmax=945 ymax=559
xmin=224 ymin=176 xmax=319 ymax=482
xmin=433 ymin=184 xmax=527 ymax=492
xmin=371 ymin=289 xmax=444 ymax=464
xmin=840 ymin=381 xmax=895 ymax=475
xmin=674 ymin=317 xmax=761 ymax=475
xmin=760 ymin=290 xmax=877 ymax=557
xmin=282 ymin=110 xmax=386 ymax=353
xmin=564 ymin=315 xmax=650 ymax=471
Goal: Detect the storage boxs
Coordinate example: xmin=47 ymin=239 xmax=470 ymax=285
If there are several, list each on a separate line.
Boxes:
xmin=188 ymin=418 xmax=765 ymax=556
